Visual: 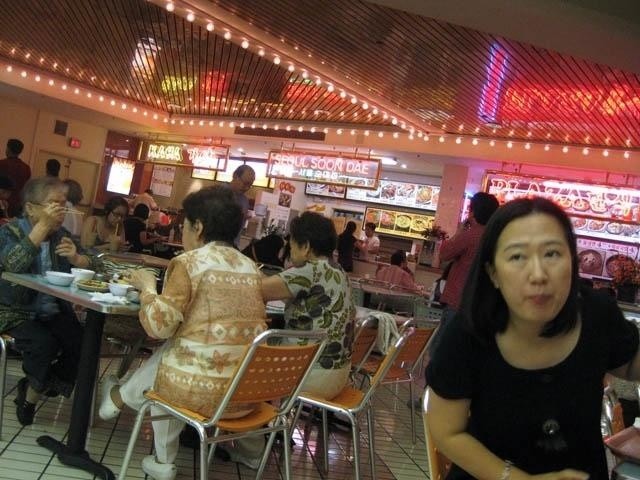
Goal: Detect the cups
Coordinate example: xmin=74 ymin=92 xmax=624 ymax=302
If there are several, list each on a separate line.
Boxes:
xmin=85 ymin=232 xmax=98 ymax=247
xmin=110 ymin=236 xmax=121 ymax=251
xmin=95 ymin=274 xmax=104 ymax=281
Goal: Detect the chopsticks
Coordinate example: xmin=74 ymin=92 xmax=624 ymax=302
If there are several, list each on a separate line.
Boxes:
xmin=46 ymin=203 xmax=86 ymax=215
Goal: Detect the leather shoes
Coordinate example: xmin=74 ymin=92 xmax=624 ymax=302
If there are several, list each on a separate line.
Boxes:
xmin=141 ymin=455 xmax=177 ymax=480
xmin=99 ymin=375 xmax=123 ymax=421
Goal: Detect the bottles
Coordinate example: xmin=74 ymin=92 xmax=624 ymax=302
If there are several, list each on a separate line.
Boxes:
xmin=168 ymin=225 xmax=175 ymax=241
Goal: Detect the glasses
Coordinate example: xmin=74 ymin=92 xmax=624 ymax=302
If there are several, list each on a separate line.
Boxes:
xmin=238 ymin=175 xmax=254 ymax=189
xmin=111 ymin=210 xmax=125 ymax=218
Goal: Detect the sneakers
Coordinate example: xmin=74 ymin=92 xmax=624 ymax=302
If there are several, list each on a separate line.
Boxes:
xmin=226 ymin=444 xmax=260 ymax=469
xmin=17 ymin=376 xmax=35 ymax=425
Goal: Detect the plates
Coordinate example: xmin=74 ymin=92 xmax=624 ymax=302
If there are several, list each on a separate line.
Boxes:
xmin=77 ymin=282 xmax=109 ymax=293
xmin=267 ymin=300 xmax=284 ymax=313
xmin=557 ymin=196 xmax=638 ymax=221
xmin=366 ymin=184 xmax=432 ymax=204
xmin=570 ymin=217 xmax=623 ymax=235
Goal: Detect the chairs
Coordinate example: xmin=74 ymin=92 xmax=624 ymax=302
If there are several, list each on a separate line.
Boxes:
xmin=1 ymin=331 xmax=100 ymax=434
xmin=111 ymin=324 xmax=330 ymax=480
xmin=351 ymin=313 xmax=441 ymax=444
xmin=345 ymin=306 xmax=385 ymax=395
xmin=257 ymin=325 xmax=417 ymax=478
xmin=421 ymin=391 xmax=457 ymax=480
xmin=600 ymin=385 xmax=630 ymax=467
xmin=412 ymin=297 xmax=445 ymax=378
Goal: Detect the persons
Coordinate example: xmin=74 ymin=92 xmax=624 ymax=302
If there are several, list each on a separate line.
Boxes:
xmin=260 ymin=209 xmax=356 ymax=441
xmin=98 ymin=184 xmax=269 ymax=480
xmin=407 ymin=190 xmax=501 ymax=411
xmin=336 ymin=220 xmax=369 ymax=274
xmin=240 ymin=234 xmax=284 ymax=267
xmin=217 ymin=164 xmax=255 ymax=251
xmin=424 ymin=197 xmax=640 ymax=480
xmin=361 ymin=222 xmax=380 ymax=250
xmin=397 ymin=249 xmax=415 ymax=282
xmin=370 ymin=252 xmax=417 ymax=309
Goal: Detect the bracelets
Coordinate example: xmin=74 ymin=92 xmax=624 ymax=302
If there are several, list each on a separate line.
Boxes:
xmin=501 ymin=460 xmax=513 ymax=480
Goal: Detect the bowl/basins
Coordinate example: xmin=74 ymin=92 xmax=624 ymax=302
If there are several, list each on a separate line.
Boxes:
xmin=108 ymin=283 xmax=128 ymax=297
xmin=578 ymin=249 xmax=603 ymax=272
xmin=605 ymin=254 xmax=635 ymax=277
xmin=46 ymin=270 xmax=74 ymax=287
xmin=366 ymin=211 xmax=429 ymax=232
xmin=71 ymin=268 xmax=96 ymax=283
